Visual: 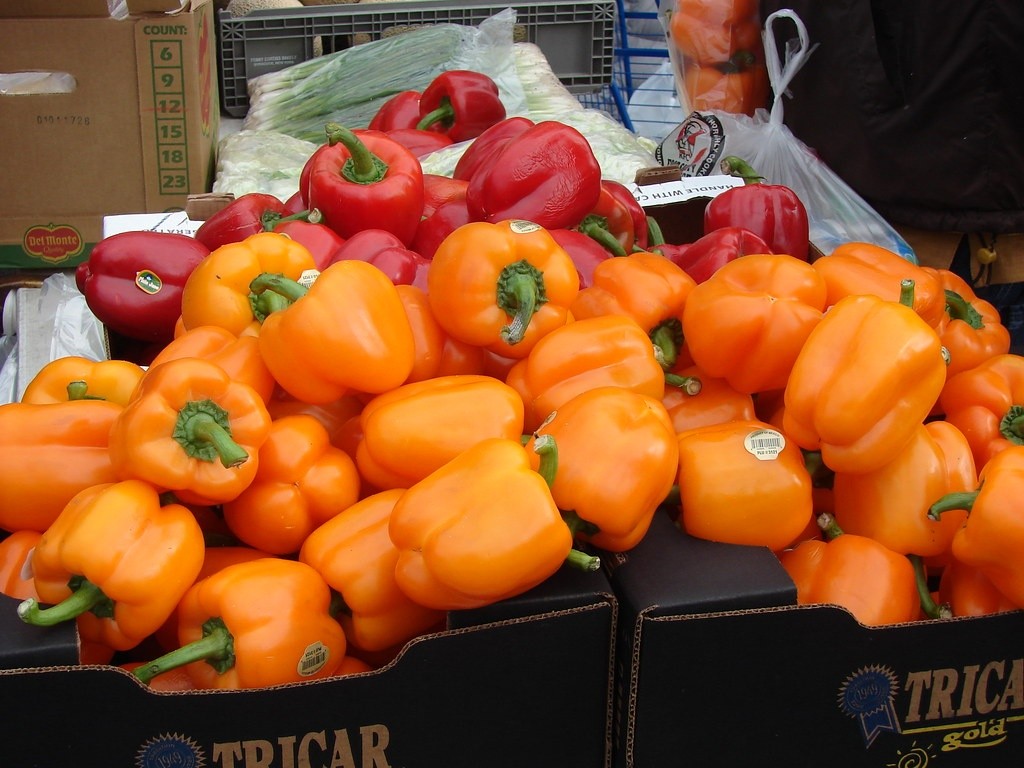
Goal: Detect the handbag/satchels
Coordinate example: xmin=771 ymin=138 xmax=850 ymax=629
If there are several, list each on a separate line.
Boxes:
xmin=657 ymin=0 xmax=769 ymax=116
xmin=653 ymin=8 xmax=919 ymax=265
xmin=627 ymin=62 xmax=685 ymax=147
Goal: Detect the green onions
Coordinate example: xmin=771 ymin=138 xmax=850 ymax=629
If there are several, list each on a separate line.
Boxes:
xmin=242 ymin=23 xmax=474 ymax=144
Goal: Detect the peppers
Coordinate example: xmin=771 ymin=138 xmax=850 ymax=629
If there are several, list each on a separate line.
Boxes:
xmin=0 ymin=72 xmax=1024 ymax=691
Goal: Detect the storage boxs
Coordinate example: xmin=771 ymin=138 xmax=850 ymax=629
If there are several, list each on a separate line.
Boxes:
xmin=219 ymin=0 xmax=621 ymax=117
xmin=0 ymin=546 xmax=622 ymax=768
xmin=0 ymin=0 xmax=225 ymax=270
xmin=622 ymin=490 xmax=1024 ymax=768
